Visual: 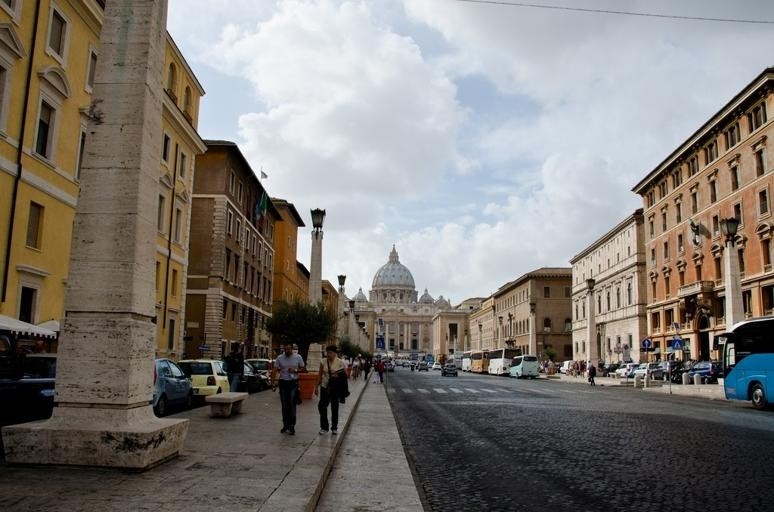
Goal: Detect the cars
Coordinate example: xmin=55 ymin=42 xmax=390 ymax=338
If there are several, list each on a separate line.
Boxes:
xmin=555 ymin=359 xmax=721 ymax=385
xmin=151 ymin=357 xmax=281 ymax=417
xmin=375 ymin=356 xmax=458 ymax=377
xmin=0 ymin=332 xmax=56 ymax=424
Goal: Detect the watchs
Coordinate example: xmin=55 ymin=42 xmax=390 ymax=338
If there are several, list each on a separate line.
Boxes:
xmin=294 ymin=368 xmax=298 ymax=373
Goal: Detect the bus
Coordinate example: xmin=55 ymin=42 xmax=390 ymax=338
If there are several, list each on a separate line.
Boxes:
xmin=711 ymin=317 xmax=773 ymax=411
xmin=453 ymin=347 xmax=540 ymax=380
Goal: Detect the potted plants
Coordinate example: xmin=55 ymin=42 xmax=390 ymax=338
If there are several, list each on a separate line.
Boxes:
xmin=337 ymin=335 xmax=361 ymax=378
xmin=542 ymin=345 xmax=560 ymax=376
xmin=263 ymin=296 xmax=337 ymax=401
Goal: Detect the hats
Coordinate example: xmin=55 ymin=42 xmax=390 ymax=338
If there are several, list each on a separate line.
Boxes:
xmin=326 ymin=346 xmax=339 ymax=352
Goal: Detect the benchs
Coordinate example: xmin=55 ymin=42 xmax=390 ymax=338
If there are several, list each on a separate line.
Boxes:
xmin=205 ymin=391 xmax=247 ymax=416
xmin=608 ymin=372 xmax=621 ymax=379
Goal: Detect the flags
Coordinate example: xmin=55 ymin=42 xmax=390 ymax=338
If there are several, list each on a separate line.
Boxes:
xmin=258 ymin=193 xmax=268 ymax=226
xmin=260 ymin=171 xmax=267 ymax=180
xmin=254 ymin=192 xmax=264 ymax=223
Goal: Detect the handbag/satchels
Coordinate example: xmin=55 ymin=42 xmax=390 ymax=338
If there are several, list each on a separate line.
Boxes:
xmin=295 ymin=388 xmax=302 ymax=404
xmin=326 ymin=377 xmax=339 ymax=396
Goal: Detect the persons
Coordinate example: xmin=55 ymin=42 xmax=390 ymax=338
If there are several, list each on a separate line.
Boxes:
xmin=270 ymin=343 xmax=305 ymax=436
xmin=340 ymin=354 xmax=373 ymax=380
xmin=223 ymin=342 xmax=245 ymax=392
xmin=538 ymin=357 xmax=592 ymax=379
xmin=637 ymin=356 xmax=718 ymax=383
xmin=314 ymin=346 xmax=345 ymax=435
xmin=376 ymin=360 xmax=385 ymax=382
xmin=589 ymin=364 xmax=596 ymax=385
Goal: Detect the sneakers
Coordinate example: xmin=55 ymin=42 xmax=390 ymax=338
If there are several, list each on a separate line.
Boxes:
xmin=319 ymin=429 xmax=337 ymax=435
xmin=281 ymin=423 xmax=295 ymax=433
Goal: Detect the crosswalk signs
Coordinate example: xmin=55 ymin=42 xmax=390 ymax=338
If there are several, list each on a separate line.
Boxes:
xmin=673 ymin=339 xmax=683 ymax=350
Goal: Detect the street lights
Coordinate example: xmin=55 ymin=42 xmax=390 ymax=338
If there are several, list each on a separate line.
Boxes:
xmin=463 ymin=329 xmax=468 ymax=351
xmin=452 ymin=333 xmax=458 ymax=355
xmin=582 ymin=277 xmax=599 ymax=370
xmin=719 ymin=216 xmax=745 ymax=332
xmin=478 ymin=324 xmax=482 ymax=351
xmin=303 ymin=208 xmax=326 ymax=375
xmin=528 ymin=302 xmax=536 ymax=355
xmin=497 ymin=315 xmax=504 ymax=349
xmin=330 ymin=275 xmax=347 ymax=371
xmin=348 ymin=300 xmax=370 ymax=369
